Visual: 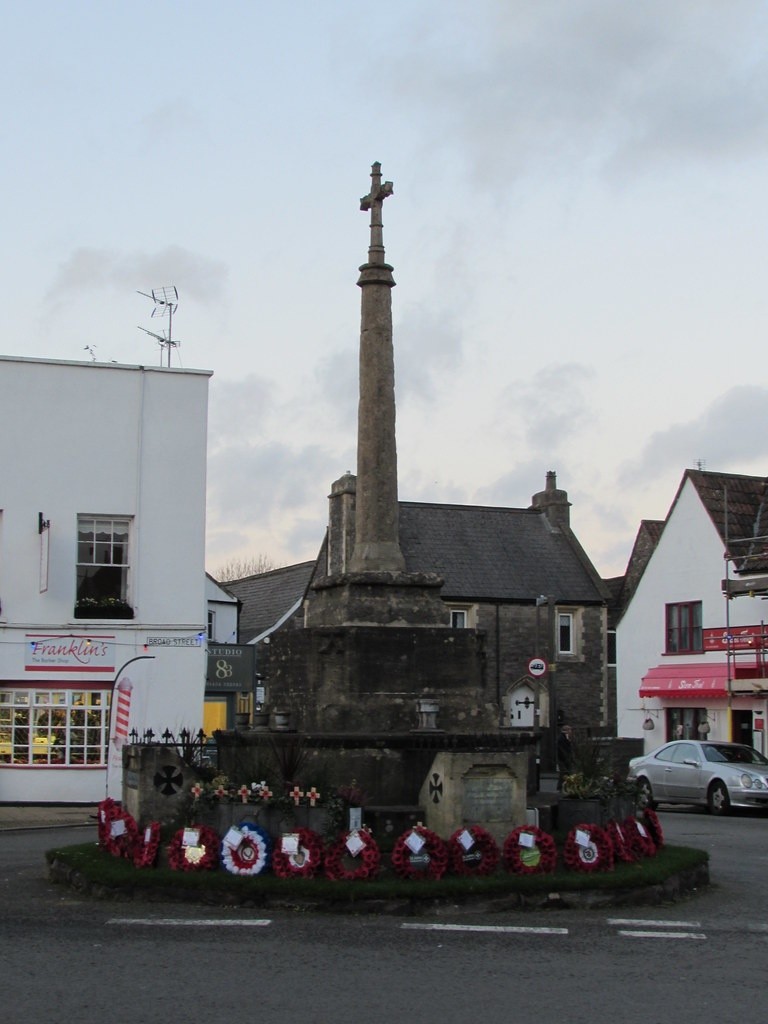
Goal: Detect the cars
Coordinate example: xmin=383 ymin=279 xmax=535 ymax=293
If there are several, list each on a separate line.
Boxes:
xmin=627 ymin=740 xmax=768 ymax=814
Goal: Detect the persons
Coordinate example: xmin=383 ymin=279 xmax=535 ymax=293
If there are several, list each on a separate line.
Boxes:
xmin=13 ymin=698 xmax=109 ymax=762
xmin=556 ymin=724 xmax=574 ymax=790
